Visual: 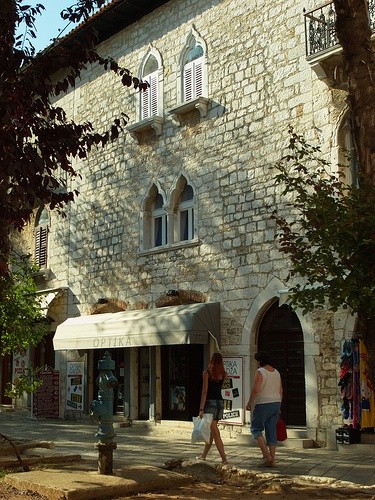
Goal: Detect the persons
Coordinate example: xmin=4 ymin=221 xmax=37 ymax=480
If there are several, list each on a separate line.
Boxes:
xmin=246 ymin=350 xmax=283 ymax=469
xmin=191 ymin=352 xmax=229 ymax=465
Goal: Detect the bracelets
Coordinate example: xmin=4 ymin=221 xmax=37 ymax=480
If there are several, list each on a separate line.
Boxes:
xmin=199 ymin=409 xmax=204 ymax=411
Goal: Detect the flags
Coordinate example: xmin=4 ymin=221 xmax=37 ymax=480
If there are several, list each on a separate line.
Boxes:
xmin=358 ymin=338 xmax=374 ymax=428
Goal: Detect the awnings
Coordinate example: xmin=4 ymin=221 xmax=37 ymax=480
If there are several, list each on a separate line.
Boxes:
xmin=50 ymin=299 xmax=220 ymax=352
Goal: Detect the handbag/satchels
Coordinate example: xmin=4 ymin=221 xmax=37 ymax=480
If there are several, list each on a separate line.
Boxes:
xmin=190 ymin=413 xmax=213 ymax=445
xmin=276 ymin=415 xmax=287 ymax=441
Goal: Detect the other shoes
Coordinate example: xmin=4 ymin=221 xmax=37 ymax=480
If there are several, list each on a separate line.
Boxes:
xmin=223 ymin=461 xmax=228 ymax=464
xmin=272 ymin=463 xmax=276 ymax=466
xmin=256 ymin=458 xmax=272 ymax=467
xmin=195 ymin=456 xmax=206 ymax=461
